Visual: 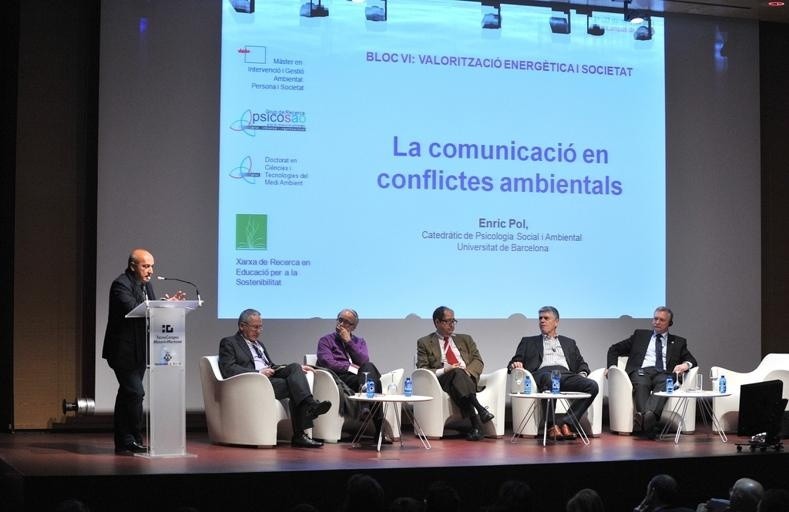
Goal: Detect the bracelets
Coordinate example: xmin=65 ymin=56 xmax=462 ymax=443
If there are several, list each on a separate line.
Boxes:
xmin=684 ymin=360 xmax=691 ymax=371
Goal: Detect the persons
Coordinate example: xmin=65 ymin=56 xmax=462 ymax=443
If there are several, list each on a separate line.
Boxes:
xmin=311 ymin=309 xmax=396 ymax=445
xmin=98 ymin=247 xmax=189 ymax=457
xmin=505 ymin=304 xmax=600 ymax=442
xmin=605 ymin=305 xmax=696 ymax=442
xmin=216 ymin=308 xmax=333 ymax=450
xmin=276 ymin=473 xmax=789 ymax=511
xmin=414 ymin=305 xmax=496 ymax=442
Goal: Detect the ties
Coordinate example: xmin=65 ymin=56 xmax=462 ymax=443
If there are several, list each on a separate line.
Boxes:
xmin=655 ymin=334 xmax=663 ymax=373
xmin=444 ymin=337 xmax=458 ymax=365
xmin=252 ymin=344 xmax=270 ymax=366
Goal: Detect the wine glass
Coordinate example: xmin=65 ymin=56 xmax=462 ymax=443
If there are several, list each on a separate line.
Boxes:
xmin=386 ymin=371 xmax=400 ymax=394
xmin=359 ymin=370 xmax=372 ymax=398
xmin=512 ymin=370 xmax=523 ymax=394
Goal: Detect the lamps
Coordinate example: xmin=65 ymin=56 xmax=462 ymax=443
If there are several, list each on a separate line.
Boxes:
xmin=226 ymin=0 xmax=654 ymax=42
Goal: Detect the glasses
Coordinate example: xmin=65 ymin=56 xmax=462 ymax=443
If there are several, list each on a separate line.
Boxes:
xmin=440 ymin=318 xmax=457 ymax=324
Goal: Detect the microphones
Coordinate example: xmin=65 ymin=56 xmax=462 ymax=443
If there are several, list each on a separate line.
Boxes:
xmin=157 ymin=276 xmax=200 ymax=300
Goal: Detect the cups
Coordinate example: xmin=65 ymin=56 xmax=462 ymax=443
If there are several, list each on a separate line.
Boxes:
xmin=681 ymin=372 xmax=705 ymax=392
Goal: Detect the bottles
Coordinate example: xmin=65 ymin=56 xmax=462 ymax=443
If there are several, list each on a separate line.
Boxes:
xmin=719 ymin=375 xmax=726 ymax=393
xmin=524 ymin=376 xmax=531 ymax=395
xmin=666 ymin=375 xmax=674 ymax=394
xmin=404 ymin=377 xmax=412 ymax=396
xmin=552 ymin=374 xmax=561 ymax=394
xmin=366 ymin=378 xmax=375 ymax=399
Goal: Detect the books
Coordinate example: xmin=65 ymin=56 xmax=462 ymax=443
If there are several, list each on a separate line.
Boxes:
xmin=560 ymin=391 xmax=583 ymax=395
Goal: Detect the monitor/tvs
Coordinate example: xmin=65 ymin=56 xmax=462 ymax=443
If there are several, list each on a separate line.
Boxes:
xmin=737 ymin=379 xmax=783 ymax=437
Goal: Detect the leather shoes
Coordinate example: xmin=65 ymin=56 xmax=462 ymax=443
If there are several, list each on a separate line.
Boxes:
xmin=548 ymin=425 xmax=565 ymax=441
xmin=478 ymin=407 xmax=494 ymax=423
xmin=115 ymin=441 xmax=147 ymax=452
xmin=376 ymin=431 xmax=393 ymax=444
xmin=360 ymin=408 xmax=371 ymax=423
xmin=291 ymin=433 xmax=323 ymax=447
xmin=304 ymin=400 xmax=331 ymax=420
xmin=561 ymin=423 xmax=578 ymax=439
xmin=644 ymin=411 xmax=656 ymax=439
xmin=466 ymin=424 xmax=485 ymax=441
xmin=633 ymin=412 xmax=642 ymax=432
